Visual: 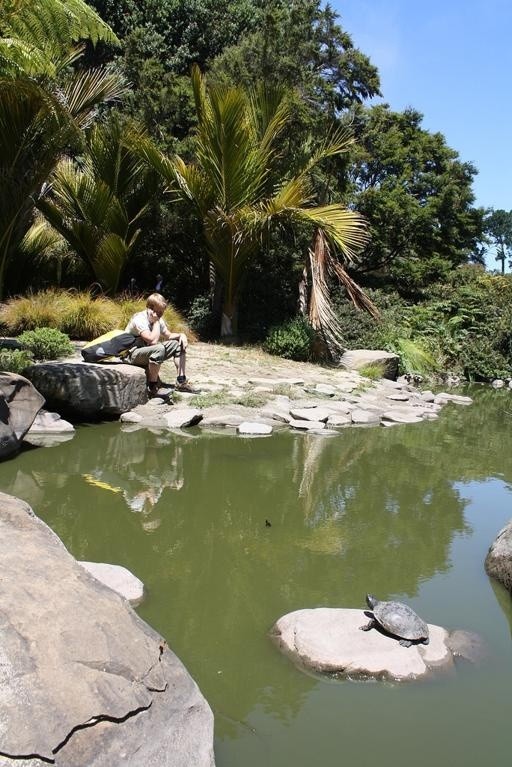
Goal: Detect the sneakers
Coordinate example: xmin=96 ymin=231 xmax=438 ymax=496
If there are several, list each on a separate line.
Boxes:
xmin=174 ymin=378 xmax=201 ymax=393
xmin=147 ymin=381 xmax=173 ymax=398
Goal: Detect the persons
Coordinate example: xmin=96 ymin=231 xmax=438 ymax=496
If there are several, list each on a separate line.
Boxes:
xmin=120 ymin=290 xmax=200 ymax=396
xmin=117 ymin=431 xmax=186 ymax=534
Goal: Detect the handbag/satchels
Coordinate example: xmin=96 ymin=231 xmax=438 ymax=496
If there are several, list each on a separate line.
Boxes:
xmin=81 ymin=330 xmax=137 ymax=364
